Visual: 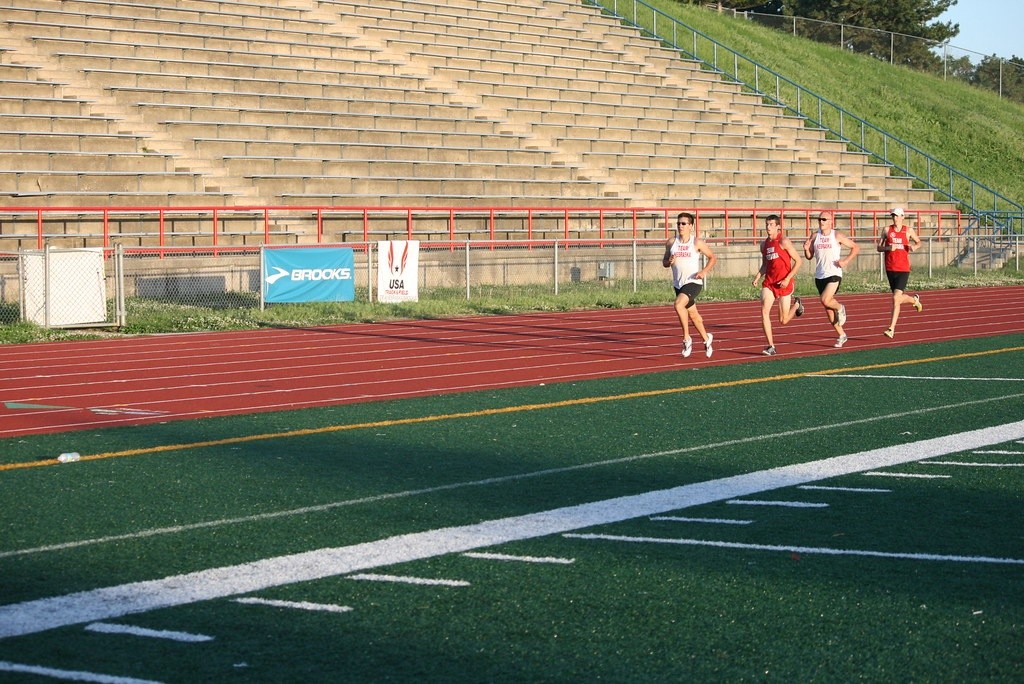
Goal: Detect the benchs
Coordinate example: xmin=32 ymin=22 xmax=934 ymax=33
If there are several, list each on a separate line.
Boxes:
xmin=0 ymin=0 xmax=1002 ymax=254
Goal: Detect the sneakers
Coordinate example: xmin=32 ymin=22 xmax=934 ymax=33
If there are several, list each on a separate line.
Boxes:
xmin=762 ymin=346 xmax=776 ymax=355
xmin=682 ymin=337 xmax=693 ymax=357
xmin=884 ymin=327 xmax=894 ymax=338
xmin=703 ymin=333 xmax=713 ymax=358
xmin=836 ymin=304 xmax=846 ymax=326
xmin=833 ymin=335 xmax=848 ymax=347
xmin=913 ymin=294 xmax=922 ymax=312
xmin=794 ymin=297 xmax=804 ymax=317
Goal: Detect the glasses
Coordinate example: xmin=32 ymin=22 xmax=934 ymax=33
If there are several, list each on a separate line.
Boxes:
xmin=817 ymin=218 xmax=831 ymax=221
xmin=677 ymin=222 xmax=690 ymax=225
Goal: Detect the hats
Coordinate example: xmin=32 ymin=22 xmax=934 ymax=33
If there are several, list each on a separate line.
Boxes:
xmin=890 ymin=207 xmax=905 ymax=216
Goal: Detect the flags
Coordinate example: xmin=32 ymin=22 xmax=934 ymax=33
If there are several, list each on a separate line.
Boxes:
xmin=260 ymin=246 xmax=356 ymax=304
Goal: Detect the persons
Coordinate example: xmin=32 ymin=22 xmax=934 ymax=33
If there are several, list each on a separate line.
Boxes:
xmin=877 ymin=207 xmax=923 ymax=338
xmin=663 ymin=212 xmax=717 ymax=359
xmin=751 ymin=214 xmax=805 ymax=356
xmin=803 ymin=209 xmax=861 ymax=348
